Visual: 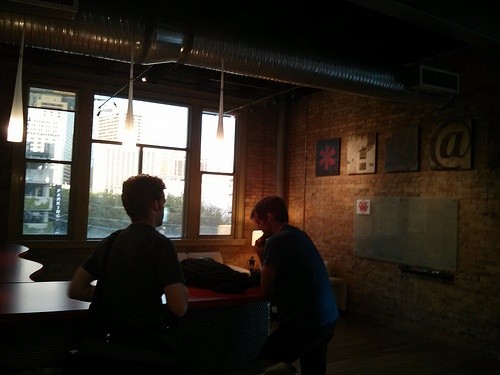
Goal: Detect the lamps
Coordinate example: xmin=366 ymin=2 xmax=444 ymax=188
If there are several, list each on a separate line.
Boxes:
xmin=214 ymin=58 xmax=226 ymax=144
xmin=122 ymin=43 xmax=138 ymax=149
xmin=6 ymin=26 xmax=26 ymax=143
xmin=252 ymin=229 xmax=269 ymax=246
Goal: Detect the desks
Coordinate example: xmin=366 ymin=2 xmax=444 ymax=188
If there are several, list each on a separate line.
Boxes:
xmin=0 ymin=243 xmax=272 ymax=375
xmin=329 ymin=276 xmax=348 ymax=312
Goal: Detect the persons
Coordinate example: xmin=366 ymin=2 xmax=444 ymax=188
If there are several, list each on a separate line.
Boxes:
xmin=249 ymin=196 xmax=339 ymax=373
xmin=66 ymin=174 xmax=188 ymax=375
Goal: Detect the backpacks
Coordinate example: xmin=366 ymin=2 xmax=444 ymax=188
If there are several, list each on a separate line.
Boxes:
xmin=179 ymin=257 xmax=250 ymax=295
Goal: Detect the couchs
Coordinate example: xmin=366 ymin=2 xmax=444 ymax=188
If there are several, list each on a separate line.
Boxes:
xmin=176 ymin=251 xmax=252 ymax=277
